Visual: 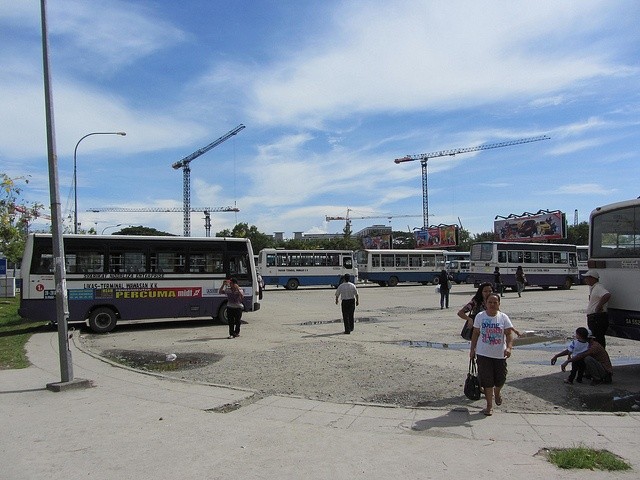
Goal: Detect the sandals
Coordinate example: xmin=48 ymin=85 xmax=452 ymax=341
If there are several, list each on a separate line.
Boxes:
xmin=494 ymin=388 xmax=502 ymax=405
xmin=484 ymin=406 xmax=493 ymax=415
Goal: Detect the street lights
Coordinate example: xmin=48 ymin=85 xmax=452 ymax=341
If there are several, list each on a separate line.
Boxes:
xmin=74 ymin=132 xmax=127 ymax=234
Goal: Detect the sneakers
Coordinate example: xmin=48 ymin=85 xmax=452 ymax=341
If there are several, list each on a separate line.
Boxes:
xmin=574 ymin=377 xmax=582 ymax=383
xmin=563 ymin=378 xmax=573 ymax=385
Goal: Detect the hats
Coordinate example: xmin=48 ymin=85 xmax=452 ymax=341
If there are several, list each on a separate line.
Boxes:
xmin=581 ymin=269 xmax=599 ymax=279
xmin=587 ymin=328 xmax=592 ymax=338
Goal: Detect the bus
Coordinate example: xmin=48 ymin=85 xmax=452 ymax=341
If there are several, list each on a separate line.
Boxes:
xmin=258 ymin=248 xmax=356 ymax=290
xmin=418 ymin=260 xmax=470 ymax=285
xmin=18 ymin=232 xmax=261 ymax=334
xmin=586 ymin=195 xmax=640 ymax=342
xmin=470 ymin=241 xmax=581 ymax=292
xmin=561 ymin=245 xmax=626 ymax=286
xmin=357 ymin=249 xmax=447 ymax=287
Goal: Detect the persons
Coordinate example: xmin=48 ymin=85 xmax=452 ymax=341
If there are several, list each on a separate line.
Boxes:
xmin=439 ymin=270 xmax=453 ymax=308
xmin=564 ymin=327 xmax=589 ymax=384
xmin=257 ymin=273 xmax=263 ymax=299
xmin=219 ymin=278 xmax=244 ymax=338
xmin=515 ymin=266 xmax=525 ymax=297
xmin=551 ymin=329 xmax=613 ymax=386
xmin=335 ymin=274 xmax=359 ymax=334
xmin=470 ymin=293 xmax=513 ymax=415
xmin=457 ymin=283 xmax=524 ymax=393
xmin=493 ymin=267 xmax=503 ymax=297
xmin=581 ymin=270 xmax=611 ymax=349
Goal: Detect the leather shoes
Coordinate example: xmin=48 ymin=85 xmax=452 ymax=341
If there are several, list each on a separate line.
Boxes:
xmin=228 ymin=335 xmax=234 ymax=339
xmin=234 ymin=333 xmax=239 ymax=337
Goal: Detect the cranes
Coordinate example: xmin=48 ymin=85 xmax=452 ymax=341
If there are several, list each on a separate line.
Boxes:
xmin=85 ymin=201 xmax=240 ymax=237
xmin=171 ymin=123 xmax=246 ymax=237
xmin=325 ymin=208 xmax=435 ymax=239
xmin=394 ymin=134 xmax=551 ymax=239
xmin=0 ymin=199 xmax=51 ymax=234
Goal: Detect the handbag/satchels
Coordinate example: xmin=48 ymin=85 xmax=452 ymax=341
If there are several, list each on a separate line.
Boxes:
xmin=461 ymin=301 xmax=480 ymax=341
xmin=464 ymin=358 xmax=481 ymax=400
xmin=447 ymin=275 xmax=452 ymax=289
xmin=518 ymin=273 xmax=527 ymax=283
